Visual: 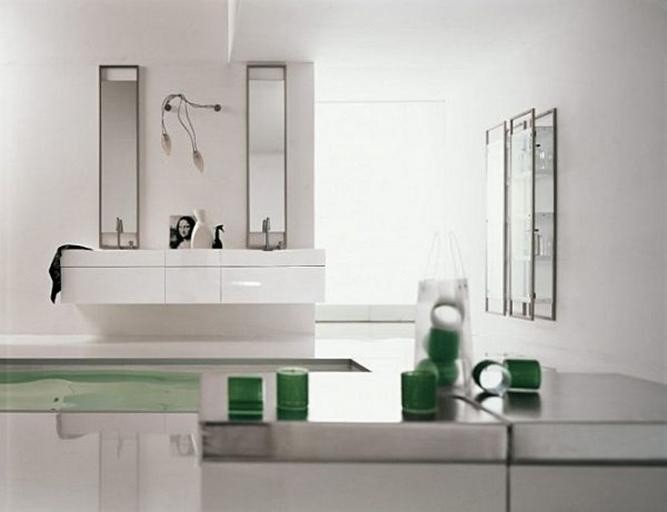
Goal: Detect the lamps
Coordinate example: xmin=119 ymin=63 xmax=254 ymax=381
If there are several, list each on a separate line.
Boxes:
xmin=532 ymin=108 xmax=557 ymax=320
xmin=483 ymin=120 xmax=507 ymax=316
xmin=245 ymin=61 xmax=288 ymax=249
xmin=97 ymin=63 xmax=141 ymax=248
xmin=507 ymin=110 xmax=532 ymax=318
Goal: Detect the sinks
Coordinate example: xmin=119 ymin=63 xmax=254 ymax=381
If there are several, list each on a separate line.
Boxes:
xmin=1 ymin=357 xmax=374 ymax=375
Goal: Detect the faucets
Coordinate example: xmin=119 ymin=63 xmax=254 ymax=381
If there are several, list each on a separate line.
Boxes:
xmin=262 ymin=220 xmax=272 ymax=251
xmin=267 ymin=217 xmax=270 ymax=230
xmin=120 ymin=220 xmax=123 ymax=231
xmin=116 ymin=217 xmax=120 ymax=249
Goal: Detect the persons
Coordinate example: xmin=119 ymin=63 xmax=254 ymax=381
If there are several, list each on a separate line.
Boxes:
xmin=169 ymin=215 xmax=195 ymax=247
